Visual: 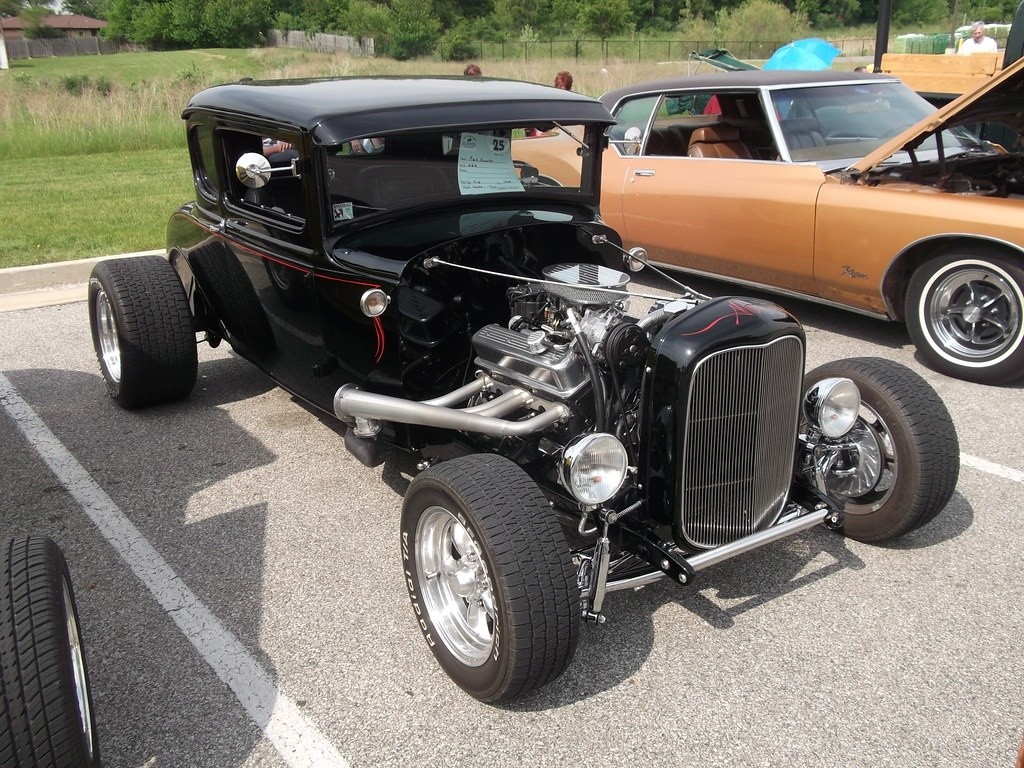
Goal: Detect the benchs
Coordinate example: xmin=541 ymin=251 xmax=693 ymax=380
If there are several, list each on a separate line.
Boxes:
xmin=649 ymin=122 xmax=770 ymax=159
xmin=336 ymin=157 xmax=457 ymax=211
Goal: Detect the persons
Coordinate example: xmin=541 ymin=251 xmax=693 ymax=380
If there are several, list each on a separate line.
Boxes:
xmin=853 ymin=66 xmax=867 ymax=73
xmin=555 ymin=71 xmax=573 ymax=91
xmin=349 ymin=138 xmax=385 ymax=153
xmin=464 ymin=65 xmax=482 ymax=77
xmin=956 ymin=21 xmax=997 ymax=56
xmin=262 ymin=137 xmax=299 ymax=164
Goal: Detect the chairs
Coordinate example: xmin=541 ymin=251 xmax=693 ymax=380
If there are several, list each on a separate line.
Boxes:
xmin=779 ymin=117 xmax=826 ymax=150
xmin=689 ymin=126 xmax=749 ymax=160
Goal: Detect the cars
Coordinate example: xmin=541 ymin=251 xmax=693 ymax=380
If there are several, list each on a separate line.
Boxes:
xmin=510 ymin=54 xmax=1024 ymax=381
xmin=88 ymin=74 xmax=960 ymax=702
xmin=852 ymin=0 xmax=1023 ymax=165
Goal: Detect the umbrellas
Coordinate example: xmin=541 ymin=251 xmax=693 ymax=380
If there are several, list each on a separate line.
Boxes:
xmin=762 ymin=38 xmax=842 ymax=71
